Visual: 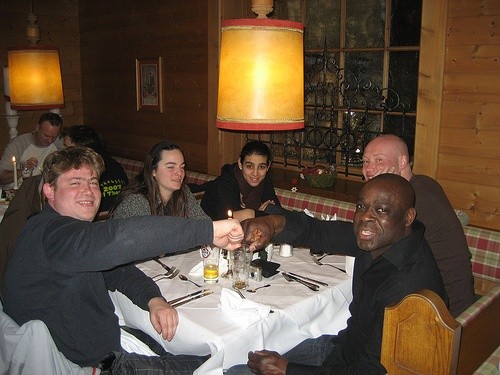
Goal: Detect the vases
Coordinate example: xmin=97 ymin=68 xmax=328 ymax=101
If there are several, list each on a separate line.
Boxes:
xmin=304 ymin=172 xmax=337 ymax=188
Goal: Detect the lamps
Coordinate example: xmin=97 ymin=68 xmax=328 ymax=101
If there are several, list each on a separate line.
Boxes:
xmin=216 ymin=0 xmax=306 ymax=132
xmin=7 ymin=0 xmax=65 ymax=110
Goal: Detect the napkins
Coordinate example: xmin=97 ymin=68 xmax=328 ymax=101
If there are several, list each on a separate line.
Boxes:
xmin=189 ymin=257 xmax=229 ymax=277
xmin=220 ymin=288 xmax=270 ymax=327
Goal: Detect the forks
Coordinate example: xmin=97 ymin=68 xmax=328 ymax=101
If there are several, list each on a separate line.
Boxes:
xmin=312 ymin=257 xmax=346 ymax=274
xmin=150 ymin=266 xmax=180 ymax=282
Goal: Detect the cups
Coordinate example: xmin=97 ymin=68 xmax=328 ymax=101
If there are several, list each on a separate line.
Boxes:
xmin=249 ymin=266 xmax=262 ymax=281
xmin=231 ymin=244 xmax=252 ymax=289
xmin=345 ymin=255 xmax=355 ymax=276
xmin=280 ymin=243 xmax=293 ymax=257
xmin=264 ymin=243 xmax=274 ymax=262
xmin=201 ymin=244 xmax=221 ymax=284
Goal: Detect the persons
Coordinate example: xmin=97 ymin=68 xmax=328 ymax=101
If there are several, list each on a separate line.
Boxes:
xmin=223 ymin=174 xmax=450 ymax=375
xmin=0 ymin=145 xmax=244 ymax=375
xmin=361 ymin=134 xmax=483 ymax=319
xmin=107 ymin=142 xmax=212 ymax=267
xmin=59 ymin=125 xmax=129 ymax=211
xmin=201 ymin=140 xmax=281 ymax=222
xmin=0 ymin=112 xmax=67 ymax=186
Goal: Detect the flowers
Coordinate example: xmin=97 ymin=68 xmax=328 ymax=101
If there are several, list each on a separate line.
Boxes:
xmin=291 ymin=165 xmax=335 ymax=193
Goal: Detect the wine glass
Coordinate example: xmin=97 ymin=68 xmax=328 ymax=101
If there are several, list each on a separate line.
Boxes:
xmin=220 ymin=247 xmax=234 ymax=279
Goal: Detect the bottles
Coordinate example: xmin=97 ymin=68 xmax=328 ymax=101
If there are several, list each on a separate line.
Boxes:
xmin=22 ymin=168 xmax=32 ymax=180
xmin=0 ymin=185 xmax=14 ymax=203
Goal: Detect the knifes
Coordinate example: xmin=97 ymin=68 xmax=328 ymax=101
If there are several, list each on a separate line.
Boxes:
xmin=153 ymin=257 xmax=174 ymax=275
xmin=288 ymin=272 xmax=328 ymax=286
xmin=282 ymin=273 xmax=319 ymax=289
xmin=168 ymin=289 xmax=210 ymax=305
xmin=172 ymin=292 xmax=214 ymax=309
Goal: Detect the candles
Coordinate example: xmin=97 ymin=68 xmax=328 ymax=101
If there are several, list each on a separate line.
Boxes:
xmin=12 ymin=156 xmax=17 ymax=187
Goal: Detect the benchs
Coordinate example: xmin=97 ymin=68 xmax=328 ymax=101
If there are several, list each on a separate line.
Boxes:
xmin=379 ymin=286 xmax=500 ymax=375
xmin=107 ymin=156 xmax=500 ymax=302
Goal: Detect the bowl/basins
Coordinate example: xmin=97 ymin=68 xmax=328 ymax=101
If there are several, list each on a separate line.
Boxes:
xmin=306 ymin=173 xmax=334 ymax=188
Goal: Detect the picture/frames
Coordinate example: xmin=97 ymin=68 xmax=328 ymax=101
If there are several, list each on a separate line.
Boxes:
xmin=135 ymin=56 xmax=163 ymax=114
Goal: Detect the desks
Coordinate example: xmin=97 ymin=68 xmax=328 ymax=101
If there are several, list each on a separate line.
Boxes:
xmin=108 ymin=244 xmax=355 ymax=375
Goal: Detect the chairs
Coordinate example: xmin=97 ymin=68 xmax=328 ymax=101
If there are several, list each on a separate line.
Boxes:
xmin=0 ymin=299 xmax=101 ymax=375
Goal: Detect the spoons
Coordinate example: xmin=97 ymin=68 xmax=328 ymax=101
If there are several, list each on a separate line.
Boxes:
xmin=247 ymin=285 xmax=271 ymax=293
xmin=179 ymin=274 xmax=202 ymax=287
